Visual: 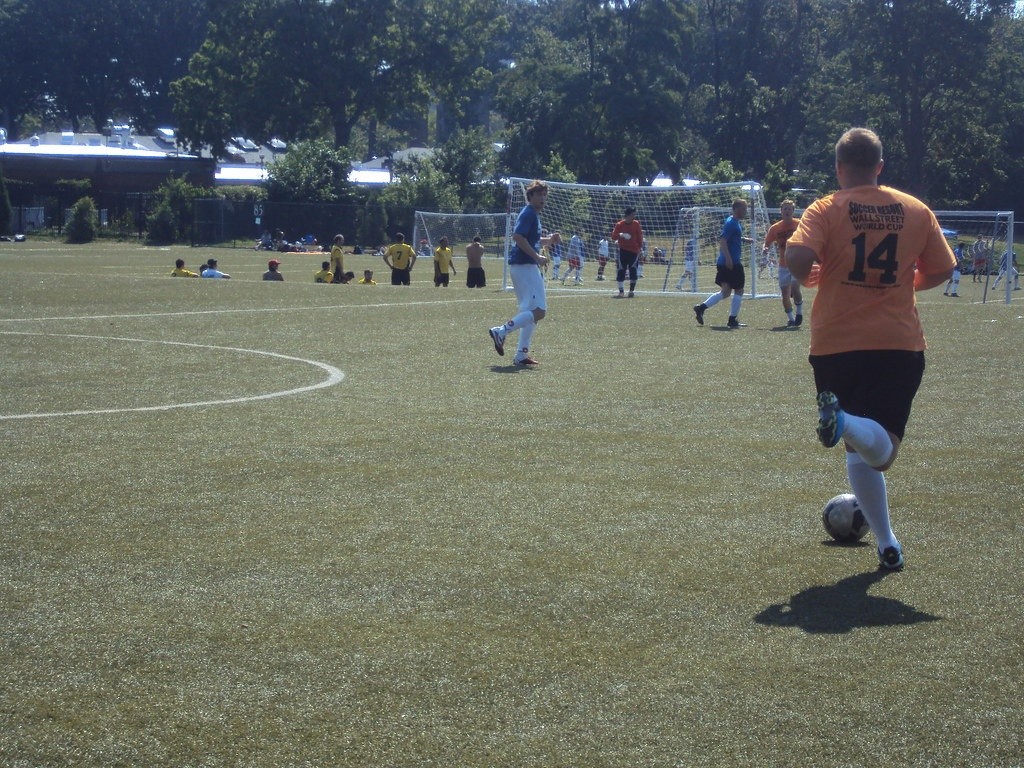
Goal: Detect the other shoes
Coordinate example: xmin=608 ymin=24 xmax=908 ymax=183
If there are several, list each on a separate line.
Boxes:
xmin=992 ymin=287 xmax=995 ymax=290
xmin=1014 ymin=287 xmax=1022 ymax=290
xmin=692 ymin=290 xmax=699 ymax=293
xmin=640 ymin=274 xmax=645 ymax=278
xmin=675 ymin=285 xmax=685 ymax=291
xmin=943 ymin=292 xmax=948 ymax=296
xmin=789 ymin=321 xmax=795 ymax=326
xmin=618 ymin=293 xmax=624 ymax=297
xmin=978 ymin=278 xmax=982 ymax=282
xmin=794 ymin=314 xmax=803 ymax=326
xmin=575 ymin=282 xmax=583 ymax=287
xmin=598 ymin=276 xmax=606 ymax=280
xmin=973 ymin=278 xmax=976 ymax=281
xmin=951 ymin=293 xmax=960 ymax=297
xmin=628 ymin=291 xmax=634 ymax=298
xmin=562 ymin=283 xmax=570 ymax=287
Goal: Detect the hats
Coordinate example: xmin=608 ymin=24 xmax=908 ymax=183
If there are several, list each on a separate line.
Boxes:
xmin=208 ymin=258 xmax=217 ymax=265
xmin=267 ymin=259 xmax=282 ymax=267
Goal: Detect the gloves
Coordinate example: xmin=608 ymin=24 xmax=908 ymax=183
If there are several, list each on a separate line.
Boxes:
xmin=623 ymin=233 xmax=631 ymax=239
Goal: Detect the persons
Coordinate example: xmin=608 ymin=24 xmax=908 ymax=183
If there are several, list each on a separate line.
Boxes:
xmin=676 ymin=233 xmax=702 ymax=291
xmin=784 ymin=128 xmax=957 ymax=571
xmin=345 ymin=245 xmax=361 ymax=255
xmin=200 ymin=258 xmax=231 ymax=278
xmin=559 ymin=227 xmax=585 ymax=286
xmin=358 ymin=269 xmax=377 ymax=285
xmin=434 ymin=236 xmax=457 ymax=287
xmin=596 ymin=233 xmax=608 ymax=279
xmin=169 ymin=259 xmax=198 ymax=278
xmin=466 ymin=236 xmax=486 ymax=288
xmin=611 ymin=207 xmax=648 ymax=297
xmin=262 ymin=228 xmax=298 ymax=252
xmin=693 ymin=199 xmax=754 ymax=327
xmin=944 ymin=242 xmax=965 ymax=297
xmin=314 ymin=261 xmax=355 ymax=283
xmin=972 ymin=234 xmax=988 ymax=283
xmin=330 ymin=234 xmax=345 ymax=274
xmin=262 ymin=259 xmax=283 ymax=281
xmin=376 ymin=232 xmax=416 ymax=285
xmin=992 ymin=245 xmax=1022 ymax=290
xmin=762 ymin=198 xmax=804 ymax=327
xmin=488 ymin=181 xmax=561 ymax=364
xmin=539 ymin=227 xmax=561 ymax=280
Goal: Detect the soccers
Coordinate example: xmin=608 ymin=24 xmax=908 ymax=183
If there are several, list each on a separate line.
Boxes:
xmin=822 ymin=493 xmax=871 ymax=543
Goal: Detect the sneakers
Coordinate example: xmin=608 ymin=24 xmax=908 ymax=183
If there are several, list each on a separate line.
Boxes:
xmin=694 ymin=304 xmax=704 ymax=324
xmin=728 ymin=320 xmax=747 ymax=329
xmin=876 ymin=547 xmax=905 ymax=572
xmin=489 ymin=327 xmax=506 ymax=356
xmin=514 ymin=353 xmax=539 ymax=368
xmin=816 ymin=391 xmax=844 ymax=448
